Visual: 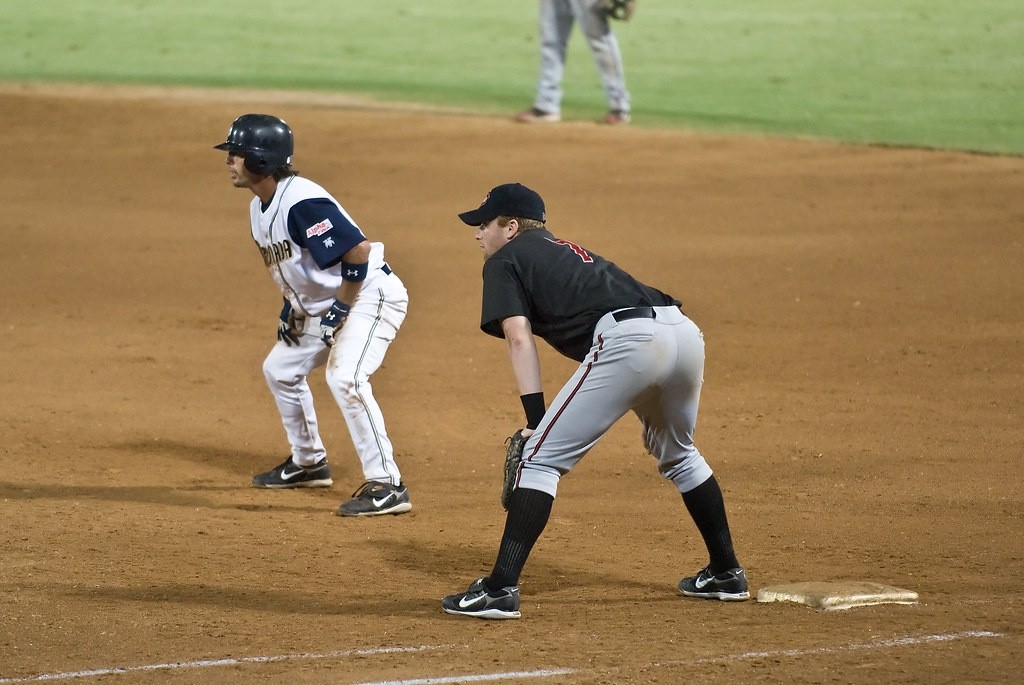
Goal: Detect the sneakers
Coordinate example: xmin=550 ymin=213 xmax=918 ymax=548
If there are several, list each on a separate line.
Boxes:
xmin=678 ymin=562 xmax=751 ymax=601
xmin=252 ymin=454 xmax=334 ymax=489
xmin=335 ymin=480 xmax=413 ymax=517
xmin=440 ymin=576 xmax=522 ymax=619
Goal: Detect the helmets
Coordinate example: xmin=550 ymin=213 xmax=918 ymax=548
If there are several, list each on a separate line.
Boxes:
xmin=213 ymin=112 xmax=294 ymax=167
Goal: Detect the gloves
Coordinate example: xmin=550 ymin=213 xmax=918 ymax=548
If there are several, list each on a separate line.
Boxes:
xmin=319 ymin=298 xmax=351 ymax=348
xmin=277 ymin=295 xmax=304 ymax=347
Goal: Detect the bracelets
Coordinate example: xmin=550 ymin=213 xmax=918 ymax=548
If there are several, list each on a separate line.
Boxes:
xmin=341 ymin=259 xmax=369 ymax=281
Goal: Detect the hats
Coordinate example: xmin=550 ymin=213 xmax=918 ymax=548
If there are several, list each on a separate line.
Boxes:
xmin=457 ymin=182 xmax=546 ymax=226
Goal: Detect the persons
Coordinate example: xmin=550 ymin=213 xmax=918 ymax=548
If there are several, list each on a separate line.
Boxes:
xmin=441 ymin=181 xmax=750 ymax=619
xmin=213 ymin=113 xmax=412 ymax=516
xmin=515 ymin=0 xmax=635 ymax=126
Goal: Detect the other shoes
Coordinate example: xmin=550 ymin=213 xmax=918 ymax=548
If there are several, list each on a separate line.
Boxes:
xmin=601 ymin=109 xmax=632 ymax=125
xmin=515 ymin=108 xmax=562 ymax=123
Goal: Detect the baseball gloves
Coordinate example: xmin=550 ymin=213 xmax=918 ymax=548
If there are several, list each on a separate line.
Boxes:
xmin=498 ymin=428 xmax=525 ymax=511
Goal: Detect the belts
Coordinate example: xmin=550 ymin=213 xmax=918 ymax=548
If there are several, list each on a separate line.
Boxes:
xmin=381 ymin=263 xmax=392 ymax=275
xmin=612 ymin=308 xmax=684 ymax=323
